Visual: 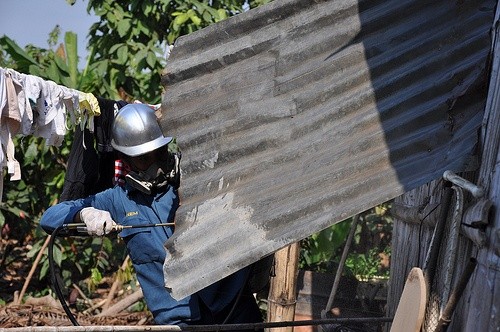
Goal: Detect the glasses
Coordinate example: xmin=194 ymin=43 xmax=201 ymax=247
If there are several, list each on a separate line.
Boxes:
xmin=137 ymin=144 xmax=169 ymax=162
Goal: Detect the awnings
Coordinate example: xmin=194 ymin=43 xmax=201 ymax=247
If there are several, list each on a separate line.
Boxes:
xmin=160 ymin=0 xmax=485 ymax=301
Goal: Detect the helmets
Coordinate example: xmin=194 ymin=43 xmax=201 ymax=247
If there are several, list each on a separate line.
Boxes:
xmin=110 ymin=103 xmax=172 ymax=158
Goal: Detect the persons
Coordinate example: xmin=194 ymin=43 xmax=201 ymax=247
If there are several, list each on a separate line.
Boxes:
xmin=39 ymin=102 xmax=275 ymax=332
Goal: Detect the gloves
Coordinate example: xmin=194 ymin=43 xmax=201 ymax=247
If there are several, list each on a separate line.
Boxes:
xmin=79 ymin=206 xmax=117 ymax=236
